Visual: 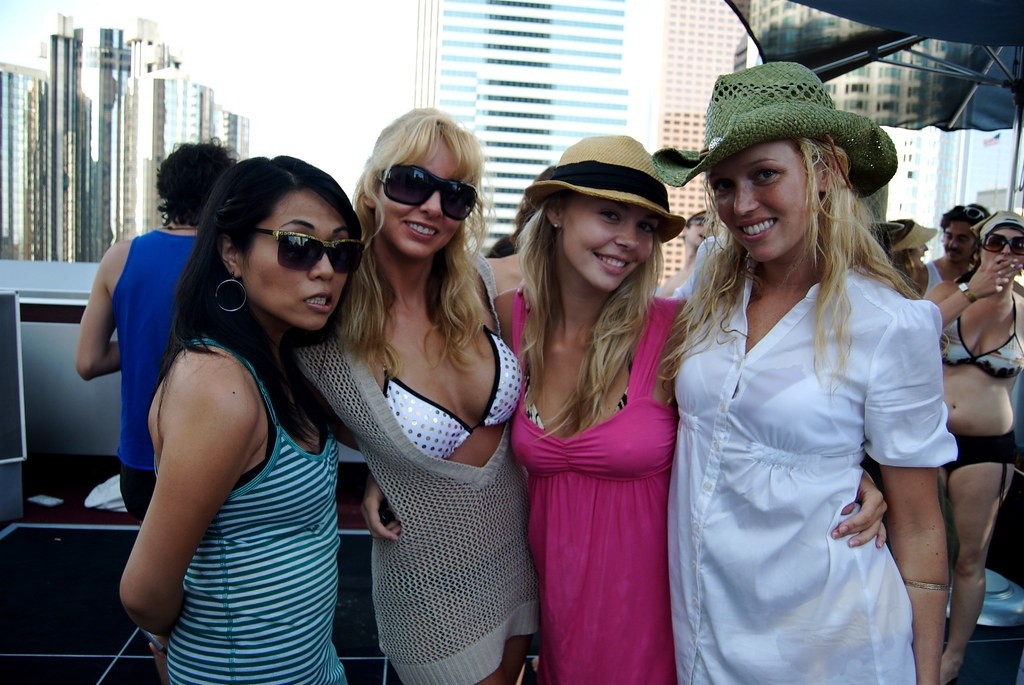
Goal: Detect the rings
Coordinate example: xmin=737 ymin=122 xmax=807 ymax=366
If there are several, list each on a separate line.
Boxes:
xmin=1010 ymin=264 xmax=1015 ymax=268
xmin=998 ymin=271 xmax=1001 ymax=276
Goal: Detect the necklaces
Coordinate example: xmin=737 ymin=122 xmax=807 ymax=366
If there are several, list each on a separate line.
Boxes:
xmin=160 ymin=226 xmax=196 ymax=229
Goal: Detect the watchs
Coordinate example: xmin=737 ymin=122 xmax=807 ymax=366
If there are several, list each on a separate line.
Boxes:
xmin=959 ymin=283 xmax=976 ymax=302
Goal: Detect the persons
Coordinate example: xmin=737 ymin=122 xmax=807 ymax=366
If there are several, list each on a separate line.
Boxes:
xmin=293 ymin=107 xmax=540 ymax=685
xmin=871 ymin=204 xmax=1024 ymax=685
xmin=119 ymin=156 xmax=363 ymax=685
xmin=75 ymin=143 xmax=235 ymax=526
xmin=359 ymin=137 xmax=888 ymax=685
xmin=655 ymin=208 xmax=718 ymax=297
xmin=485 ymin=166 xmax=555 ymax=293
xmin=652 ymin=61 xmax=959 ymax=685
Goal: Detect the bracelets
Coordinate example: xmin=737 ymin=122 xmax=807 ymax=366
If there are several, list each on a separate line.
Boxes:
xmin=903 ymin=579 xmax=949 ymax=591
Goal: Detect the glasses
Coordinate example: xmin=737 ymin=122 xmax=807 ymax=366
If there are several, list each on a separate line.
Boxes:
xmin=953 ymin=205 xmax=985 ymax=219
xmin=251 ymin=227 xmax=366 ymax=273
xmin=689 ymin=217 xmax=714 ymax=227
xmin=376 ymin=164 xmax=478 ymax=221
xmin=978 ymin=233 xmax=1024 ymax=255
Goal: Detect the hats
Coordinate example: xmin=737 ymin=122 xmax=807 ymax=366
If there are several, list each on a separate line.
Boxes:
xmin=888 ymin=219 xmax=938 ymax=252
xmin=970 ymin=210 xmax=1024 ymax=239
xmin=523 ymin=135 xmax=686 ymax=244
xmin=651 ymin=61 xmax=898 ymax=198
